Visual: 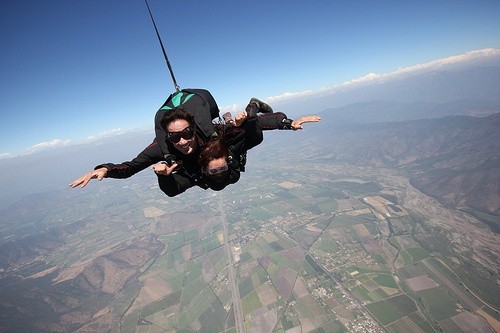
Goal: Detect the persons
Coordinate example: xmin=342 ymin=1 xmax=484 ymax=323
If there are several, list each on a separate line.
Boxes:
xmin=151 ymin=96 xmax=323 ymax=198
xmin=68 ymin=88 xmax=233 ymax=189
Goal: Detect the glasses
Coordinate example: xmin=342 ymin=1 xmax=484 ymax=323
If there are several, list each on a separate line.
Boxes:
xmin=168 ymin=123 xmax=195 ymax=144
